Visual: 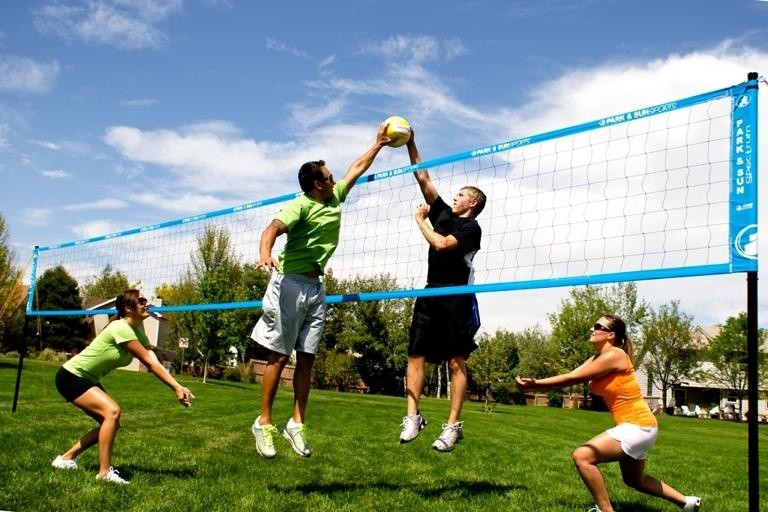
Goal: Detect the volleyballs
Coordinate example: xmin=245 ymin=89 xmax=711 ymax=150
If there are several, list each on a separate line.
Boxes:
xmin=385 ymin=116 xmax=412 ymax=148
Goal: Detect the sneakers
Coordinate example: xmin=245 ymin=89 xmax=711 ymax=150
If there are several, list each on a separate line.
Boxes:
xmin=683 ymin=494 xmax=701 ymax=512
xmin=52 ymin=456 xmax=79 ymax=471
xmin=283 ymin=419 xmax=310 ymax=457
xmin=399 ymin=412 xmax=427 ymax=442
xmin=252 ymin=415 xmax=276 ymax=458
xmin=432 ymin=424 xmax=462 ymax=453
xmin=96 ymin=471 xmax=126 ymax=483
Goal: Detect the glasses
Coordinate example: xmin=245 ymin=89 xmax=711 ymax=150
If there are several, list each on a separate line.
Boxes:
xmin=137 ymin=297 xmax=149 ymax=303
xmin=594 ymin=323 xmax=611 ymax=332
xmin=324 ymin=175 xmax=333 ymax=181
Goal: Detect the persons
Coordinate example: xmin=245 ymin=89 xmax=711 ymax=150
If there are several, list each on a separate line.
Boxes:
xmin=50 ymin=289 xmax=197 ymax=486
xmin=249 ymin=122 xmax=393 ymax=459
xmin=399 ymin=126 xmax=486 ymax=454
xmin=513 ymin=314 xmax=701 ymax=512
